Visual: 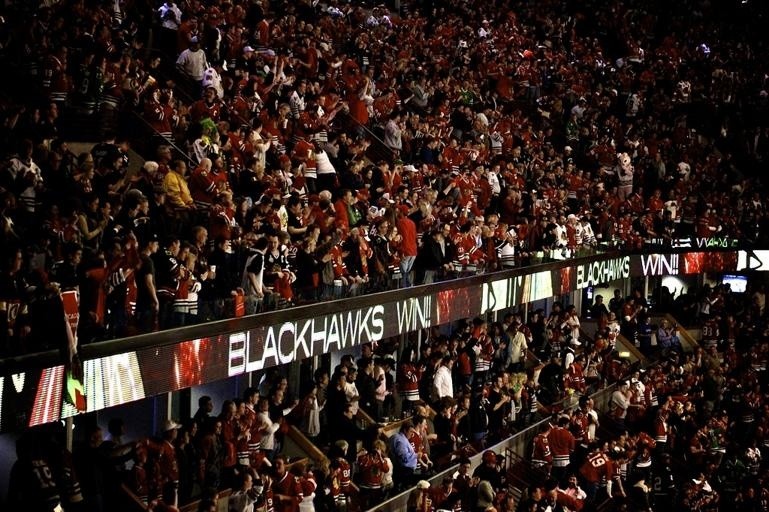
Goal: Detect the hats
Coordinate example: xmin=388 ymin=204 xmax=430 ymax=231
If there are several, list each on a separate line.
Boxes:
xmin=630 ymin=377 xmax=639 ymax=385
xmin=164 ymin=421 xmax=183 ymax=430
xmin=570 ymin=338 xmax=581 ymax=345
xmin=568 ymin=214 xmax=578 ymax=219
xmin=417 ymin=480 xmax=430 ymax=489
xmin=402 ymin=165 xmax=419 ymax=173
